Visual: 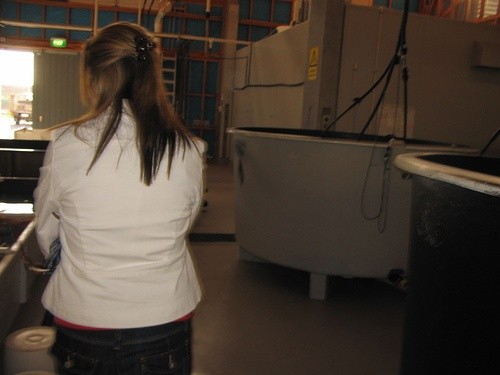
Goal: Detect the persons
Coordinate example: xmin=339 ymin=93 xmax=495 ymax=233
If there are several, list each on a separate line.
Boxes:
xmin=34 ymin=21 xmax=207 ymax=375
